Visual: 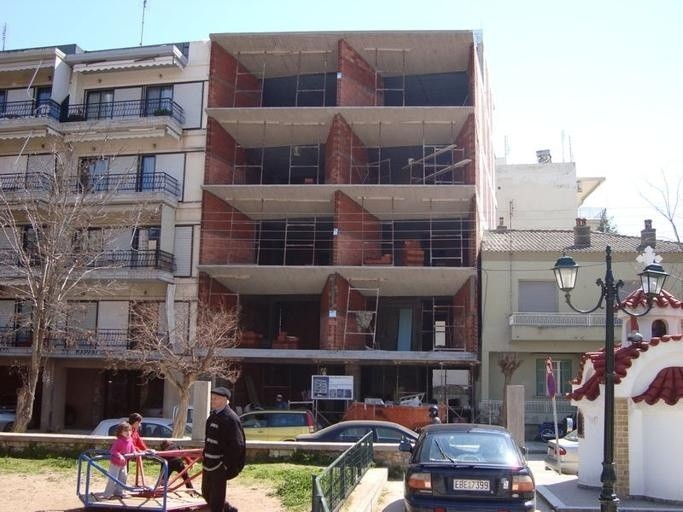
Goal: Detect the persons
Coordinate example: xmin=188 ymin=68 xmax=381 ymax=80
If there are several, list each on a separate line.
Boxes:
xmin=103 ymin=422 xmax=138 ymax=500
xmin=202 ymin=387 xmax=246 ymax=512
xmin=159 ymin=440 xmax=193 ymax=497
xmin=429 ymin=399 xmax=442 ymax=424
xmin=127 ymin=413 xmax=151 ymax=473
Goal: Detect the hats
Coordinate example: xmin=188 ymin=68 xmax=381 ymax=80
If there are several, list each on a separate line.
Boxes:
xmin=211 ymin=387 xmax=231 ymax=399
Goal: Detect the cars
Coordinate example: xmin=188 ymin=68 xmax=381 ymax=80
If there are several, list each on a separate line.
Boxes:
xmin=545 ymin=428 xmax=580 ymax=476
xmin=90 ymin=417 xmax=191 ymax=441
xmin=1 ymin=409 xmax=17 ymax=433
xmin=398 ymin=422 xmax=536 ymax=512
xmin=297 ymin=418 xmax=419 ymax=443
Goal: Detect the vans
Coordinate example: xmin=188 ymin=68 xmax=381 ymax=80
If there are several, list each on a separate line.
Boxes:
xmin=236 ymin=409 xmax=316 ymax=443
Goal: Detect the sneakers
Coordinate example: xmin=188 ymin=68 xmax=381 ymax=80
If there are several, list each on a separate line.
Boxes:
xmin=104 ymin=493 xmax=127 ymax=499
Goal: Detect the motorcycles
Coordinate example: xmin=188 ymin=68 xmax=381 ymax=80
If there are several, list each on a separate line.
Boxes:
xmin=541 ymin=421 xmax=563 ymax=444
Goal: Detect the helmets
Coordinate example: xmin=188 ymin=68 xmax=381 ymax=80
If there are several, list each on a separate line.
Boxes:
xmin=429 ymin=405 xmax=439 ymax=417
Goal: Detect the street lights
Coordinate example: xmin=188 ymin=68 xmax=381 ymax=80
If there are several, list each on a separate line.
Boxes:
xmin=551 ymin=244 xmax=668 ymax=511
